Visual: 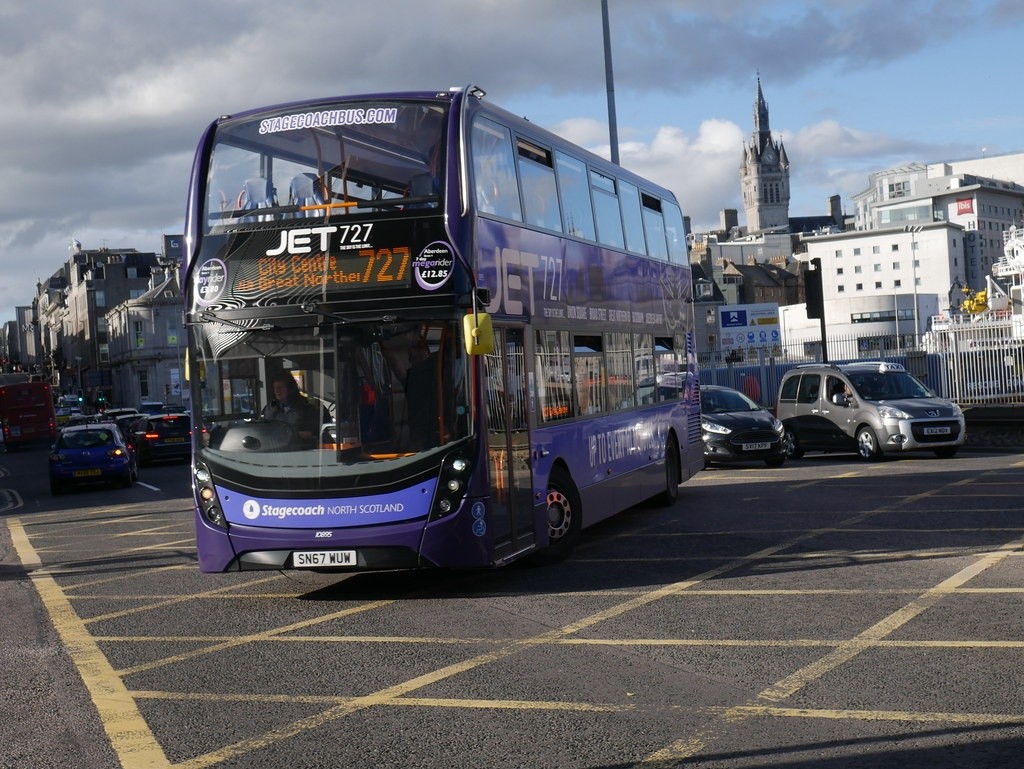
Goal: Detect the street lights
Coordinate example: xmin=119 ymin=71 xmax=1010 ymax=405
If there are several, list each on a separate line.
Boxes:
xmin=904 ymin=224 xmax=925 ymax=352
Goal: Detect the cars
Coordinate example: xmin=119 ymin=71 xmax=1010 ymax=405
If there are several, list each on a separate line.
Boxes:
xmin=48 ymin=407 xmax=192 ymax=494
xmin=700 ymin=385 xmax=786 ymax=468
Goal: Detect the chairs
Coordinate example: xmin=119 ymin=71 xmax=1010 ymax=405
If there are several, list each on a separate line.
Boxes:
xmin=809 ymin=383 xmax=819 ymax=398
xmin=403 ymin=173 xmax=434 ymax=208
xmin=232 ymin=173 xmax=324 ymax=221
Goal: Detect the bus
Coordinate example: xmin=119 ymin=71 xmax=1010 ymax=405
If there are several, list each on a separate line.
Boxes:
xmin=0 ymin=382 xmax=58 ymax=452
xmin=176 ymin=84 xmax=709 ymax=576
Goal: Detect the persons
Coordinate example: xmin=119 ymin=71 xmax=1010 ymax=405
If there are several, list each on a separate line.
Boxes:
xmin=258 ymin=369 xmax=319 ymax=441
xmin=407 ymin=142 xmax=443 ymax=209
xmin=841 ymin=382 xmax=856 ymax=403
xmin=378 ymin=333 xmax=444 ymax=452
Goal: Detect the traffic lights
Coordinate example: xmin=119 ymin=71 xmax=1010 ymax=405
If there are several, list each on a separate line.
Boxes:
xmin=98 ymin=391 xmax=102 ymax=402
xmin=103 ymin=392 xmax=105 ymax=401
xmin=78 ymin=389 xmax=83 ymax=402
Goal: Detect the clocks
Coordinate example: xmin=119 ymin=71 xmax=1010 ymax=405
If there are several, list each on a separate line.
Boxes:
xmin=766 ymin=152 xmax=774 ymax=161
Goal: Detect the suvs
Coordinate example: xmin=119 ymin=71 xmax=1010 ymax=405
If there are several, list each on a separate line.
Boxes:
xmin=775 ymin=361 xmax=967 ymax=461
